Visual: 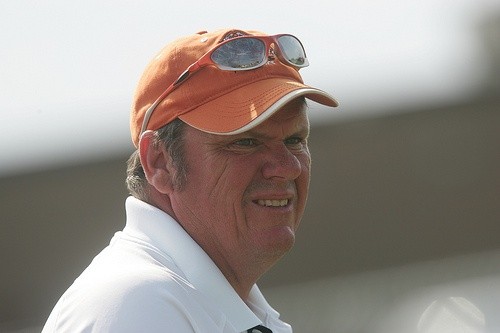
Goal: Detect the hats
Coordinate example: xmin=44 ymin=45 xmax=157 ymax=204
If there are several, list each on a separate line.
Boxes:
xmin=130 ymin=30 xmax=339 ymax=151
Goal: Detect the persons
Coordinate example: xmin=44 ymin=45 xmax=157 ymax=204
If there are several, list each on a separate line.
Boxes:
xmin=41 ymin=30 xmax=339 ymax=333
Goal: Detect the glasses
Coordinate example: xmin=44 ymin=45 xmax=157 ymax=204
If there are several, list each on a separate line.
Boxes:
xmin=138 ymin=33 xmax=310 ymax=139
